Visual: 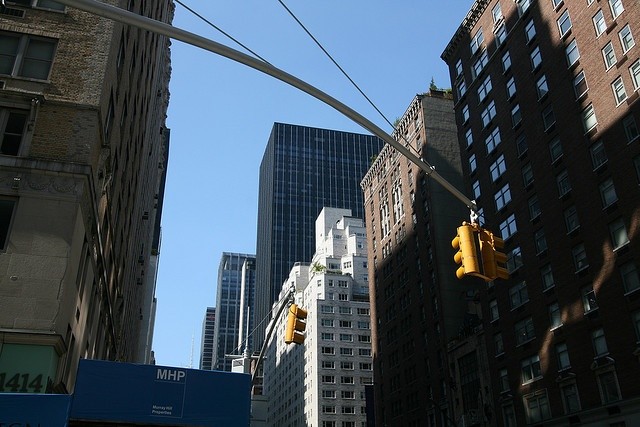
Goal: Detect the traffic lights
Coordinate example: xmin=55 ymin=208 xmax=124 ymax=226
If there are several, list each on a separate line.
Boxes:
xmin=285 ymin=304 xmax=307 ymax=345
xmin=452 ymin=226 xmax=479 ymax=279
xmin=479 ymin=231 xmax=509 ymax=278
